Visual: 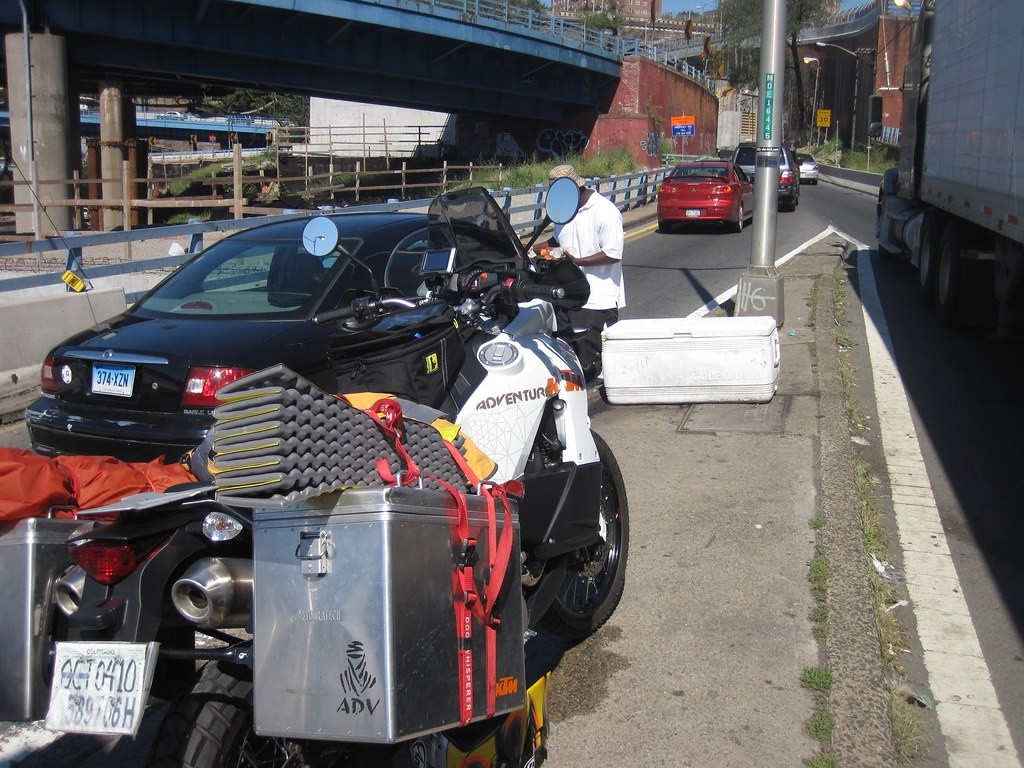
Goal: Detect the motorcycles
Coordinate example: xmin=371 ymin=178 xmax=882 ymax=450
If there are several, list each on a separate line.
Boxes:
xmin=44 ymin=175 xmax=637 ymax=765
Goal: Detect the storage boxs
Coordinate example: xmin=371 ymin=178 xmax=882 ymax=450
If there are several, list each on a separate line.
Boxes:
xmin=0 ymin=505 xmax=115 ymax=721
xmin=601 ymin=316 xmax=780 ymax=404
xmin=253 ymin=470 xmax=526 ymax=744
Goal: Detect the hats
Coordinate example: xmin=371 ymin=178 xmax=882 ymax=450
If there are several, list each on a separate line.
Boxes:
xmin=548 ymin=164 xmax=585 ymax=188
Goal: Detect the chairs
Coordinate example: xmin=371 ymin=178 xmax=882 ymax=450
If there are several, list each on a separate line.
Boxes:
xmin=719 ymin=171 xmax=727 ymax=176
xmin=287 ymin=253 xmax=324 ymax=286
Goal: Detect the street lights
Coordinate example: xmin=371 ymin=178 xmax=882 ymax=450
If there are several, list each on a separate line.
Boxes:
xmin=816 ymin=41 xmax=858 ymax=158
xmin=802 ymin=57 xmax=820 ymax=153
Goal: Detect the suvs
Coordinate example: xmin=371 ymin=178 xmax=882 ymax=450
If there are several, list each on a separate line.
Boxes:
xmin=729 ymin=141 xmax=803 ymax=213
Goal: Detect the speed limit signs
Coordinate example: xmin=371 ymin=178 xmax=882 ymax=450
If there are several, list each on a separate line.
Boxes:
xmin=816 ymin=110 xmax=831 ymax=129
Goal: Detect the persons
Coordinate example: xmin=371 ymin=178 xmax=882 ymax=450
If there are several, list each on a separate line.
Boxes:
xmin=532 ymin=164 xmax=626 ymax=378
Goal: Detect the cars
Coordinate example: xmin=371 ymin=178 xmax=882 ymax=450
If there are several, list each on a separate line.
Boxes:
xmin=21 ymin=202 xmax=598 ymax=463
xmin=657 ymin=162 xmax=753 ymax=233
xmin=796 ymin=153 xmax=820 ymax=185
